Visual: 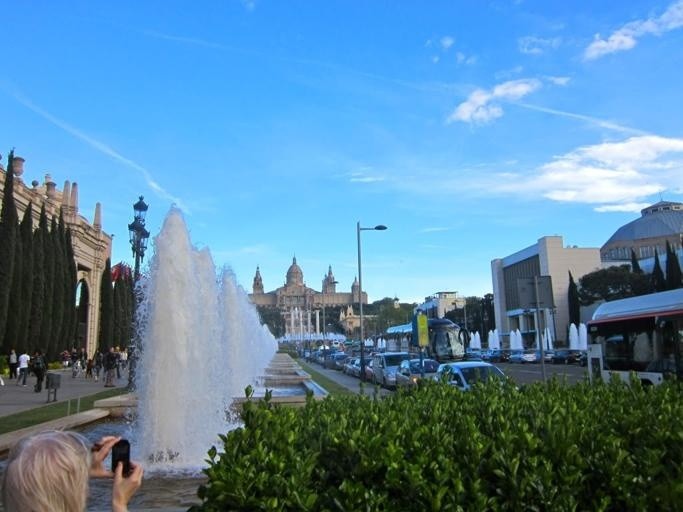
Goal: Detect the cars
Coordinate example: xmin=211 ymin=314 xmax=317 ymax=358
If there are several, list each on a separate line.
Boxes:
xmin=435 ymin=362 xmax=520 ymax=391
xmin=396 ymin=359 xmax=439 ymax=386
xmin=343 ymin=357 xmax=373 ymax=382
xmin=467 ymin=348 xmax=586 ymax=367
xmin=304 ymin=349 xmax=347 ymax=371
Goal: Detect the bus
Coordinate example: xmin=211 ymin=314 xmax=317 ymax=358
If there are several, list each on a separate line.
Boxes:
xmin=587 ymin=288 xmax=683 ymax=388
xmin=374 ymin=319 xmax=471 ymax=362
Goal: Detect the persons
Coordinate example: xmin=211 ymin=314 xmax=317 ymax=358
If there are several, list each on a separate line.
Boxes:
xmin=1 ymin=427 xmax=144 ymax=512
xmin=0 ymin=344 xmax=128 ymax=392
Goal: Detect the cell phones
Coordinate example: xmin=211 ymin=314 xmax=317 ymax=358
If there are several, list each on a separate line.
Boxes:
xmin=112 ymin=439 xmax=130 ymax=475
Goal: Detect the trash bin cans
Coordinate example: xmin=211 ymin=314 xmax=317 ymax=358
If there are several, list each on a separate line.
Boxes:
xmin=46 ymin=373 xmax=60 ymax=389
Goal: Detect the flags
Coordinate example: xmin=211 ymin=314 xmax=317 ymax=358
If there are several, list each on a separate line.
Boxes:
xmin=111 ymin=260 xmax=147 ymax=288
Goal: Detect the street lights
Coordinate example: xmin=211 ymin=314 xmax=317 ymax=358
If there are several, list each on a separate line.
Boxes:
xmin=356 ymin=222 xmax=387 ymax=383
xmin=129 ymin=194 xmax=150 ymax=396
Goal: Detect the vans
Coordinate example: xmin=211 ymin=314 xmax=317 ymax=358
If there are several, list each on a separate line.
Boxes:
xmin=373 ymin=352 xmax=410 ymax=389
